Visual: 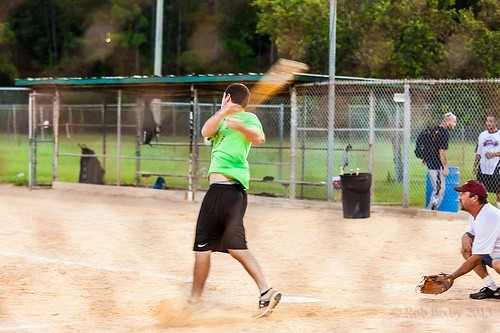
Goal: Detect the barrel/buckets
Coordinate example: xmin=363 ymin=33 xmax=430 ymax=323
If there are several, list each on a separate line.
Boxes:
xmin=339 ymin=173 xmax=372 ymax=218
xmin=425 ymin=167 xmax=459 ymax=213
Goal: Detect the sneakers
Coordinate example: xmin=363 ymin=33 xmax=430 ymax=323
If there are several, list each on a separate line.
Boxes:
xmin=469 ymin=287 xmax=500 ymax=300
xmin=252 ymin=288 xmax=282 ymax=318
xmin=182 ymin=299 xmax=207 ymax=314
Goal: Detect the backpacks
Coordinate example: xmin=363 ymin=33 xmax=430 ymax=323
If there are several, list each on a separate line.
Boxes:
xmin=414 ymin=126 xmax=445 ymax=159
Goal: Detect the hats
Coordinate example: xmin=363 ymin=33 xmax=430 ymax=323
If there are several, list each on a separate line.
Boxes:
xmin=453 ymin=180 xmax=488 ymax=198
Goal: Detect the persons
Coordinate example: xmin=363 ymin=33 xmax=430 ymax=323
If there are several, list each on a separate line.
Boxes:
xmin=425 ymin=113 xmax=456 ymax=210
xmin=187 ymin=83 xmax=282 ymax=319
xmin=44 ymin=120 xmax=49 ymax=134
xmin=471 ymin=115 xmax=500 ymax=210
xmin=419 ymin=179 xmax=500 ymax=300
xmin=144 ymin=123 xmax=160 ymax=147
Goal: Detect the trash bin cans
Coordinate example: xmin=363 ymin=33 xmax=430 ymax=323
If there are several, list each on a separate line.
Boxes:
xmin=425 ymin=166 xmax=460 ymax=213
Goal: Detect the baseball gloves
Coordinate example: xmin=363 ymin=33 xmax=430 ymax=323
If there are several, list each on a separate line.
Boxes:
xmin=421 ymin=272 xmax=454 ymax=295
